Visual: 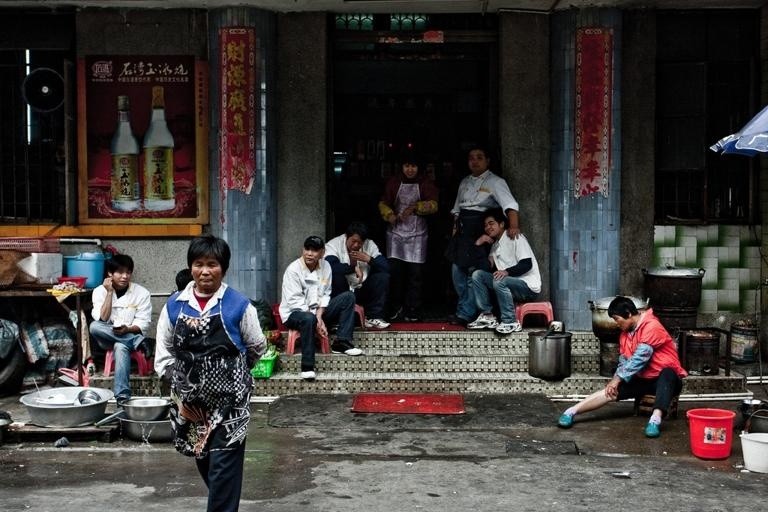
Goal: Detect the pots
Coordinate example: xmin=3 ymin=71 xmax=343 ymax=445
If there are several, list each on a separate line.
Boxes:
xmin=586 ymin=292 xmax=651 ymax=340
xmin=528 ymin=330 xmax=573 ymax=380
xmin=641 ymin=263 xmax=704 ymax=312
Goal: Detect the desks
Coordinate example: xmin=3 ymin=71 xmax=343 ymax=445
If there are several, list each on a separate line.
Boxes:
xmin=0 ymin=288 xmax=94 ymax=386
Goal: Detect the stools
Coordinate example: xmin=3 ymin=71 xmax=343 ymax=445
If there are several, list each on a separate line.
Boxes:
xmin=513 ymin=298 xmax=555 ymax=326
xmin=633 ymin=394 xmax=680 ymax=420
xmin=100 ymin=347 xmax=154 ymax=377
xmin=354 ymin=303 xmax=365 ymax=329
xmin=287 ymin=328 xmax=329 ymax=355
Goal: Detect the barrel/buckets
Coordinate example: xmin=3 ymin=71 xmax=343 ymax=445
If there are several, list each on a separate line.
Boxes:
xmin=739 ymin=410 xmax=768 ymax=475
xmin=685 ymin=408 xmax=736 ymax=461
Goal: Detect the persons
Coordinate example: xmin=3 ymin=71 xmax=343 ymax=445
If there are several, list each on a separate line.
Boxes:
xmin=378 ymin=155 xmax=439 ymax=322
xmin=466 ymin=208 xmax=542 ymax=333
xmin=559 ymin=296 xmax=688 ymax=436
xmin=89 ymin=253 xmax=152 ymax=409
xmin=323 ymin=223 xmax=391 ymax=332
xmin=176 ymin=269 xmax=193 ymax=291
xmin=445 ymin=144 xmax=521 ymax=324
xmin=279 ymin=236 xmax=362 ymax=379
xmin=153 ymin=234 xmax=268 ymax=512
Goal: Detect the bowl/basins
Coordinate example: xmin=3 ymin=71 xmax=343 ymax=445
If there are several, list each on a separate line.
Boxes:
xmin=740 ymin=401 xmax=767 ymax=432
xmin=16 ymin=386 xmax=176 ymax=441
xmin=738 ymin=433 xmax=768 ymax=474
xmin=56 ymin=277 xmax=89 ymax=290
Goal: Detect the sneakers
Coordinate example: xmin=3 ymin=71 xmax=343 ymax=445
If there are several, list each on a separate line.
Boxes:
xmin=558 ymin=412 xmax=576 ymax=427
xmin=331 ymin=341 xmax=364 ymax=357
xmin=364 ymin=317 xmax=391 ymax=331
xmin=301 ymin=370 xmax=316 ymax=380
xmin=404 ymin=309 xmax=420 ymax=322
xmin=495 ymin=320 xmax=522 ymax=335
xmin=389 ymin=304 xmax=403 ymax=320
xmin=645 ymin=421 xmax=663 ymax=437
xmin=141 ymin=338 xmax=158 ymax=360
xmin=468 ymin=314 xmax=498 ymax=331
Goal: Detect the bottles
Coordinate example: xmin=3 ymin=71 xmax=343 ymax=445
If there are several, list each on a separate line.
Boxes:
xmin=142 ymin=84 xmax=179 ymax=212
xmin=108 ymin=93 xmax=140 ymax=213
xmin=85 ymin=358 xmax=97 ymax=377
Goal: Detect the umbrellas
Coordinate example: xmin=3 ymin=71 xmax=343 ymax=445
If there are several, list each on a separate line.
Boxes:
xmin=710 ymin=105 xmax=768 ymax=158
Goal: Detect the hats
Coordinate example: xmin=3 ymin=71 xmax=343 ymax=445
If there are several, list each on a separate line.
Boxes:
xmin=304 ymin=236 xmax=325 ymax=250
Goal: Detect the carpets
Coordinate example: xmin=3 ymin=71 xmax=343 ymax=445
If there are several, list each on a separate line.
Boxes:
xmin=367 ymin=322 xmax=466 ymax=331
xmin=350 ymin=394 xmax=466 ymax=415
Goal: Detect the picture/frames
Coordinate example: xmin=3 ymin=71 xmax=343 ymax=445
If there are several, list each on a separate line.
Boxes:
xmin=77 ymin=52 xmax=210 ymax=225
